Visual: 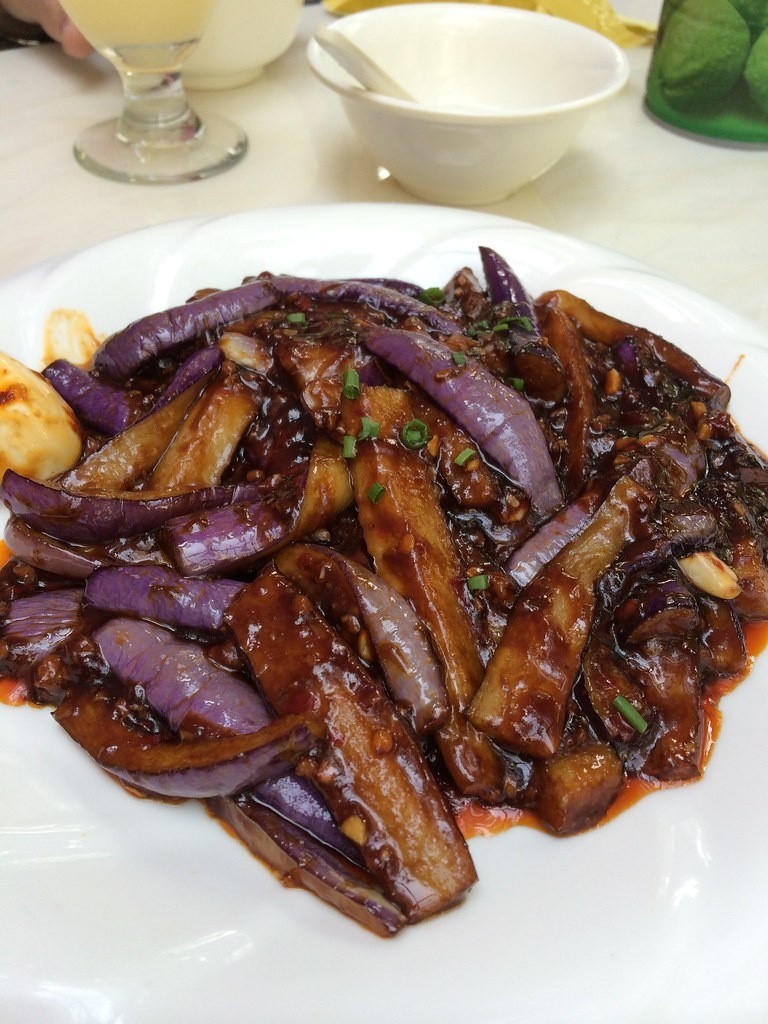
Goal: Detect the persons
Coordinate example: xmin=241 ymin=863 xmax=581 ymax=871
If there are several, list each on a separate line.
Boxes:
xmin=0 ymin=0 xmax=96 ymax=59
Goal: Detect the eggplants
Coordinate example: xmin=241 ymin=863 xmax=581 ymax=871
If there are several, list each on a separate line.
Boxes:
xmin=1 ymin=245 xmax=768 ymax=934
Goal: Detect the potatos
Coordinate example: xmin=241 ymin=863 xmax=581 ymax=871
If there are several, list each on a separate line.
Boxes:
xmin=0 ymin=349 xmax=84 ymax=479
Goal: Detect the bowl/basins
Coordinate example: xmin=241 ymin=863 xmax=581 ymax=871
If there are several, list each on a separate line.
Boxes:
xmin=182 ymin=0 xmax=303 ymax=90
xmin=306 ymin=3 xmax=631 ymax=203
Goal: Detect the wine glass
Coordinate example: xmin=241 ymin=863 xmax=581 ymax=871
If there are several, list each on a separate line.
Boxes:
xmin=57 ymin=0 xmax=250 ymax=184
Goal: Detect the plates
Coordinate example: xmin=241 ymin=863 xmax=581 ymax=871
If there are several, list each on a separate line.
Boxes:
xmin=0 ymin=202 xmax=768 ymax=1024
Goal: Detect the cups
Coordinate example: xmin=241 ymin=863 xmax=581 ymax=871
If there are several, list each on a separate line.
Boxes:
xmin=646 ymin=0 xmax=768 ymax=142
